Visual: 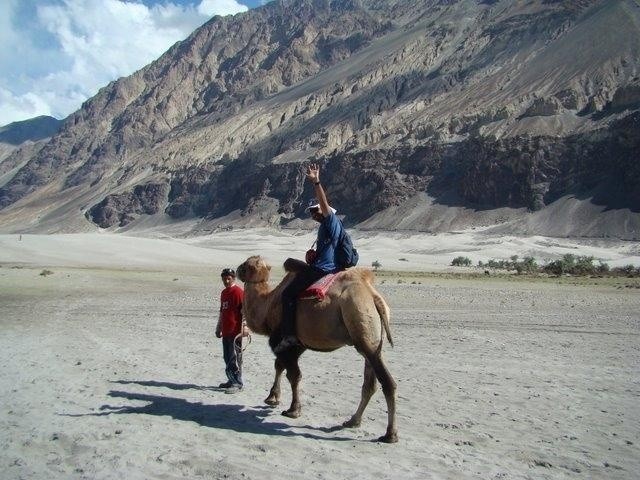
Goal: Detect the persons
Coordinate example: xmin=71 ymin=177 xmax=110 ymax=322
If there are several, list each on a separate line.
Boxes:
xmin=215 ymin=269 xmax=248 ymax=393
xmin=274 ymin=163 xmax=344 ymax=354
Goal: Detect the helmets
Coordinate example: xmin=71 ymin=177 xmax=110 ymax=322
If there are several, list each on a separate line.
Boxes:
xmin=222 ymin=269 xmax=235 ymax=274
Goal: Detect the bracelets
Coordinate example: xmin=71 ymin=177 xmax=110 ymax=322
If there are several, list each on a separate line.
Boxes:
xmin=313 ymin=180 xmax=322 ymax=186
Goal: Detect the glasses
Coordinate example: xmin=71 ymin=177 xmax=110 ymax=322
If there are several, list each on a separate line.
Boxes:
xmin=309 ymin=208 xmax=318 ymax=212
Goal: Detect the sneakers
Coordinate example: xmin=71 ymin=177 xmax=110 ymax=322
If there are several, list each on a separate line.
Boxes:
xmin=220 ymin=381 xmax=242 ymax=394
xmin=274 ymin=335 xmax=298 ymax=354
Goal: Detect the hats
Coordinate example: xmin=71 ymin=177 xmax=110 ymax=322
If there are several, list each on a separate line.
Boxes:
xmin=304 ymin=199 xmax=320 ymax=216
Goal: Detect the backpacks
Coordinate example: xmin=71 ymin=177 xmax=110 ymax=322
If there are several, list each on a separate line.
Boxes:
xmin=325 ymin=214 xmax=358 ymax=268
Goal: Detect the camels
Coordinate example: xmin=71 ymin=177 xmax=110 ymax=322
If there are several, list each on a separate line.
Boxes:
xmin=234 ymin=254 xmax=399 ymax=443
